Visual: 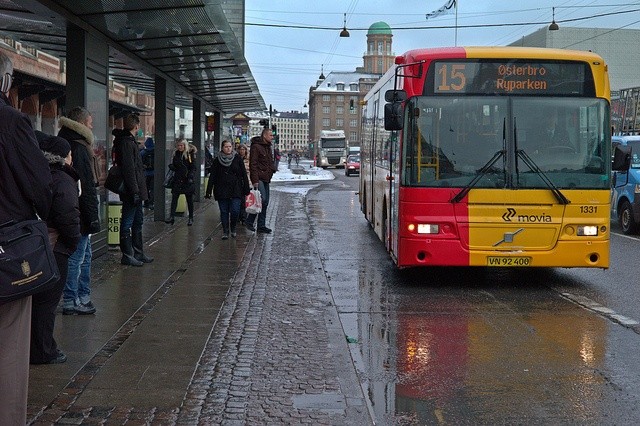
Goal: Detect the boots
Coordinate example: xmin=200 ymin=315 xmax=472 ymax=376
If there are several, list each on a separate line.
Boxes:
xmin=120 ymin=235 xmax=144 ymax=266
xmin=132 ymin=231 xmax=154 ymax=262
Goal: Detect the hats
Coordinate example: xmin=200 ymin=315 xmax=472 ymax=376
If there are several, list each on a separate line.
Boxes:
xmin=43 ymin=135 xmax=71 ymax=157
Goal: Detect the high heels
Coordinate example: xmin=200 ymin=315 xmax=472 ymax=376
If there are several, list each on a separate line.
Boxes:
xmin=188 ymin=216 xmax=193 ymax=225
xmin=166 ymin=214 xmax=174 ymax=224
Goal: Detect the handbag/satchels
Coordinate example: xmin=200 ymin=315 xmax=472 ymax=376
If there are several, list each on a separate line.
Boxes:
xmin=104 ymin=165 xmax=126 ymax=194
xmin=162 ymin=168 xmax=180 ymax=187
xmin=245 ymin=185 xmax=262 ymax=213
xmin=0 ymin=216 xmax=62 ymax=301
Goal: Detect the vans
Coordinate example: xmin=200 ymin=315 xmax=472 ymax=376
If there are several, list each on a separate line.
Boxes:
xmin=348 ymin=147 xmax=360 ymax=154
xmin=613 ymin=136 xmax=640 ymax=234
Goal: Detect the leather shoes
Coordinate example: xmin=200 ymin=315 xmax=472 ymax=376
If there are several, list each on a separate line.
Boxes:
xmin=63 ymin=303 xmax=96 ymax=314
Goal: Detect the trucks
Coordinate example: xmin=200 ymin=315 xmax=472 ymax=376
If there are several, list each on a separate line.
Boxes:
xmin=317 ymin=130 xmax=347 ymax=168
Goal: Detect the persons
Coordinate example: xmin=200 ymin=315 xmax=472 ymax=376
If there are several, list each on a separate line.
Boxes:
xmin=274 ymin=146 xmax=281 ymax=171
xmin=111 ymin=116 xmax=155 ymax=267
xmin=238 ymin=145 xmax=253 ymax=190
xmin=244 ymin=128 xmax=277 ymax=233
xmin=29 ymin=137 xmax=81 ymax=364
xmin=204 ymin=140 xmax=250 ymax=240
xmin=55 ymin=107 xmax=95 ymax=316
xmin=526 ymin=106 xmax=576 ymax=155
xmin=140 ymin=138 xmax=155 ymax=210
xmin=163 ymin=138 xmax=197 ymax=227
xmin=235 ymin=142 xmax=240 ymax=150
xmin=0 ymin=50 xmax=54 ymax=426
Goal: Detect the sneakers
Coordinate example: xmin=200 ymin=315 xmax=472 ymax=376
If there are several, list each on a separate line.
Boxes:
xmin=52 ymin=353 xmax=67 ymax=363
xmin=245 ymin=219 xmax=255 ymax=230
xmin=257 ymin=226 xmax=272 ymax=232
xmin=231 ymin=231 xmax=237 ymax=237
xmin=221 ymin=233 xmax=229 ymax=238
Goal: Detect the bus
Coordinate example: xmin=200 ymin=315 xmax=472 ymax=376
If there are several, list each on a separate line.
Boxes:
xmin=359 ymin=48 xmax=611 ymax=273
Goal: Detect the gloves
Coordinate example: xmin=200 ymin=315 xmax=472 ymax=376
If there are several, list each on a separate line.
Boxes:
xmin=89 ymin=220 xmax=100 ymax=233
xmin=132 ymin=193 xmax=140 ymax=206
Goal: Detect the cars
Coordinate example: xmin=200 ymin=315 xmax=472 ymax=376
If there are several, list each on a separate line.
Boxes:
xmin=345 ymin=155 xmax=360 ymax=176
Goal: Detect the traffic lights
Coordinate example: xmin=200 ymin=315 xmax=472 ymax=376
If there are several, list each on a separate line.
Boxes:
xmin=349 ymin=99 xmax=354 ymax=110
xmin=275 ymin=135 xmax=279 ymax=144
xmin=272 ymin=124 xmax=276 ymax=143
